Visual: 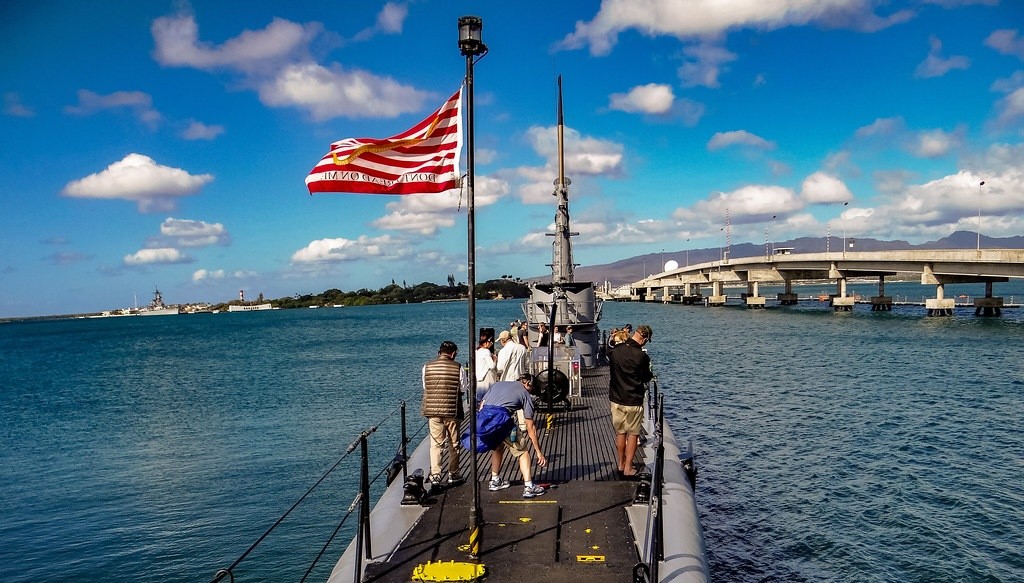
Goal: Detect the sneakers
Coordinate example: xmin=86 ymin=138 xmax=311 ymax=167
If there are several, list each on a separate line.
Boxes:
xmin=523 ymin=483 xmax=545 ymax=498
xmin=431 ymin=474 xmax=441 ymax=485
xmin=488 ymin=477 xmax=510 ymax=490
xmin=448 ymin=471 xmax=464 ymax=483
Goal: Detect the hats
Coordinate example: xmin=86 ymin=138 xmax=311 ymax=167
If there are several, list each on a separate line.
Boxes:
xmin=644 ymin=325 xmax=653 ymax=343
xmin=495 ymin=330 xmax=511 ymax=342
xmin=566 ymin=325 xmax=573 ymax=329
xmin=478 ymin=335 xmax=493 ymax=344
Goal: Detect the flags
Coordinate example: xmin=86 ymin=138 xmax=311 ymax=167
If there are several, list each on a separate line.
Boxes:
xmin=303 ymin=89 xmax=465 ymax=195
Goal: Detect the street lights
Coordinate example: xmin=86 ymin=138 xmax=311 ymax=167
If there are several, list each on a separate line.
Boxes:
xmin=687 ymin=239 xmax=690 ymax=266
xmin=844 ymin=202 xmax=847 ymax=252
xmin=977 ymin=181 xmax=984 ymax=249
xmin=772 ymin=216 xmax=776 ymax=255
xmin=719 ymin=228 xmax=723 ymax=260
xmin=662 ymin=249 xmax=664 ymax=272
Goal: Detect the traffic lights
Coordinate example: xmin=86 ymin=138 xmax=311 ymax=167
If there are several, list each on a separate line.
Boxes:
xmin=139 ymin=284 xmax=179 ymax=315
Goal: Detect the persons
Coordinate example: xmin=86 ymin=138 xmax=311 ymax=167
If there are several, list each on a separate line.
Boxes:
xmin=422 ymin=341 xmax=468 ymax=490
xmin=609 ymin=326 xmax=653 ymax=478
xmin=495 ymin=331 xmax=527 ymax=432
xmin=509 ymin=321 xmax=564 ymax=349
xmin=564 ymin=326 xmax=583 ymax=380
xmin=607 ymin=324 xmax=632 ymax=348
xmin=474 ymin=334 xmax=496 ymax=403
xmin=477 ymin=372 xmax=547 ymax=498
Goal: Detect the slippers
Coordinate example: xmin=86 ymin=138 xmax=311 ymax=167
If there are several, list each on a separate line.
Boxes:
xmin=617 ymin=463 xmax=641 ymax=481
